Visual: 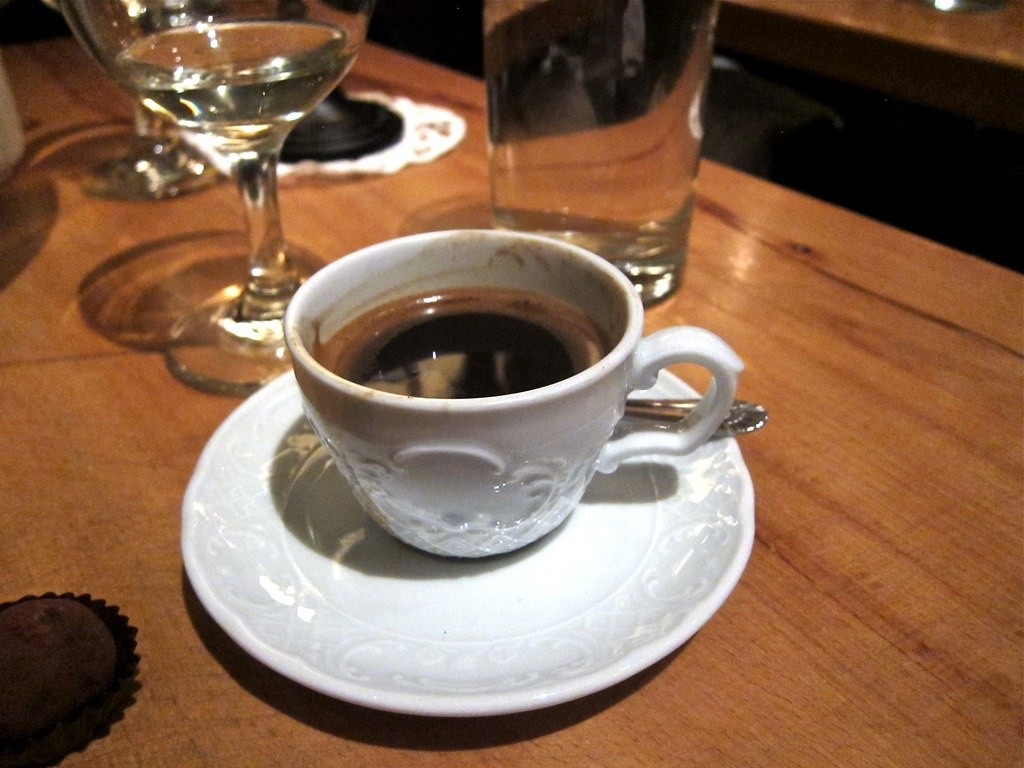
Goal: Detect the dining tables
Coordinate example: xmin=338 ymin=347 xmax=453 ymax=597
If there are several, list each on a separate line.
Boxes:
xmin=0 ymin=36 xmax=1024 ymax=767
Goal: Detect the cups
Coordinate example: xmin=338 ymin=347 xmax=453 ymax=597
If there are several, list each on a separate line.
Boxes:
xmin=284 ymin=229 xmax=743 ymax=560
xmin=484 ymin=0 xmax=717 ymax=307
xmin=277 ymin=76 xmax=400 ymax=160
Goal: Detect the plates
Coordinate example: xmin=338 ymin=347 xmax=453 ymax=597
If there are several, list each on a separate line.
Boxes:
xmin=182 ymin=366 xmax=756 ymax=716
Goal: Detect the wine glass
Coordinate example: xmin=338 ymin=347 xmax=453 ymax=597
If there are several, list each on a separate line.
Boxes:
xmin=55 ymin=0 xmax=370 ymax=393
xmin=80 ymin=104 xmax=220 ymax=198
